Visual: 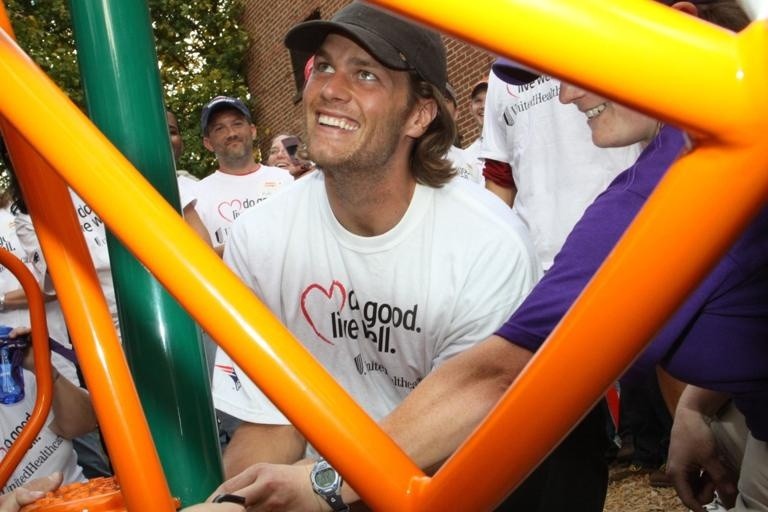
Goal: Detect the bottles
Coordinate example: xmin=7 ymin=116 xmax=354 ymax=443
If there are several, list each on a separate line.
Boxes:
xmin=0 ymin=323 xmax=27 ymax=406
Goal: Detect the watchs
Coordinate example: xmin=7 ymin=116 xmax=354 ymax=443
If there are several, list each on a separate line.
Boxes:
xmin=310 ymin=457 xmax=348 ymax=511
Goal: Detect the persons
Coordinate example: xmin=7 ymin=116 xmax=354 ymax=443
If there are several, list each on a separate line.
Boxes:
xmin=1 ymin=96 xmax=311 ymax=512
xmin=212 ymin=0 xmax=546 ymax=510
xmin=441 ymin=59 xmax=690 ymax=486
xmin=181 ymin=1 xmax=767 ymax=512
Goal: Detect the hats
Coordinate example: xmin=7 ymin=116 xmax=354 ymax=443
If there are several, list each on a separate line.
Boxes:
xmin=284 ymin=2 xmax=447 ymax=94
xmin=202 ymin=96 xmax=250 ymax=133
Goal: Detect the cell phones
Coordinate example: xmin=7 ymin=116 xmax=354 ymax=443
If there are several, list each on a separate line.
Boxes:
xmin=281 ymin=135 xmax=311 ymax=168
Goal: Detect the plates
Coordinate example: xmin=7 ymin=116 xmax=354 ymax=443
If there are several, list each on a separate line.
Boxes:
xmin=17 ymin=478 xmax=136 ymax=512
